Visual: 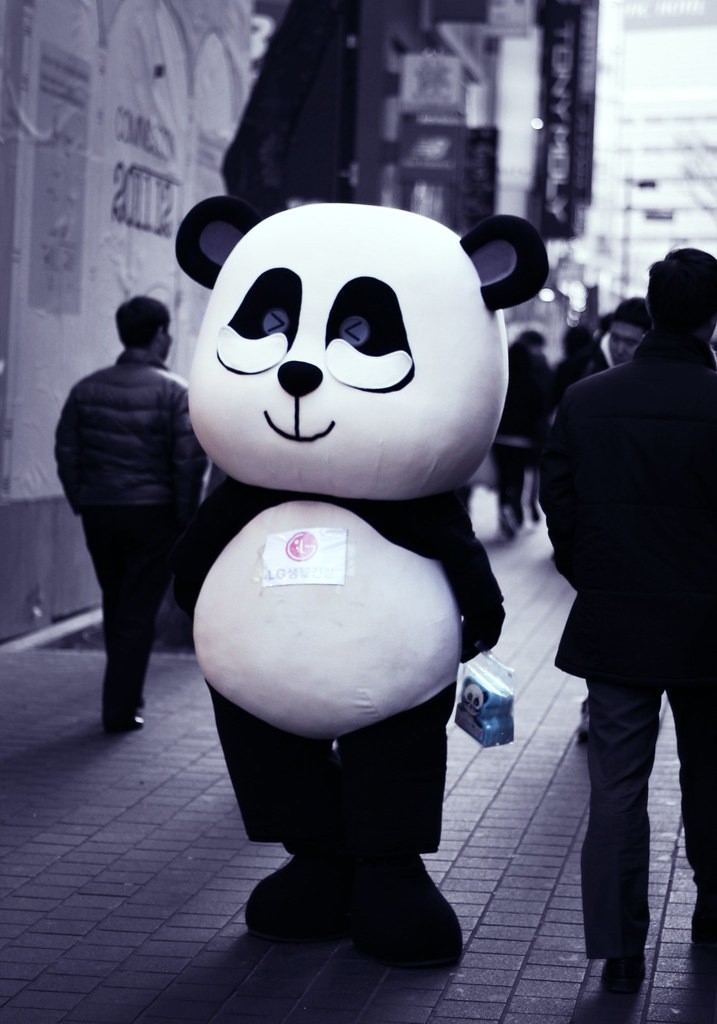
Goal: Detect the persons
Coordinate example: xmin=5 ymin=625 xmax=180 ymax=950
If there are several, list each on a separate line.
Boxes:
xmin=539 ymin=246 xmax=715 ymax=1018
xmin=53 ymin=291 xmax=209 ymax=738
xmin=486 ymin=296 xmax=661 ymax=540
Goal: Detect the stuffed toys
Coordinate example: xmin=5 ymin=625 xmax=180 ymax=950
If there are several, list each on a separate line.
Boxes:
xmin=160 ymin=190 xmax=548 ymax=978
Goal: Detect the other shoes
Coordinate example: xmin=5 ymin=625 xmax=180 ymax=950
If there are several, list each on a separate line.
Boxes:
xmin=498 ymin=495 xmax=523 ymax=540
xmin=604 ymin=952 xmax=645 ymax=994
xmin=102 ymin=716 xmax=144 ymax=732
